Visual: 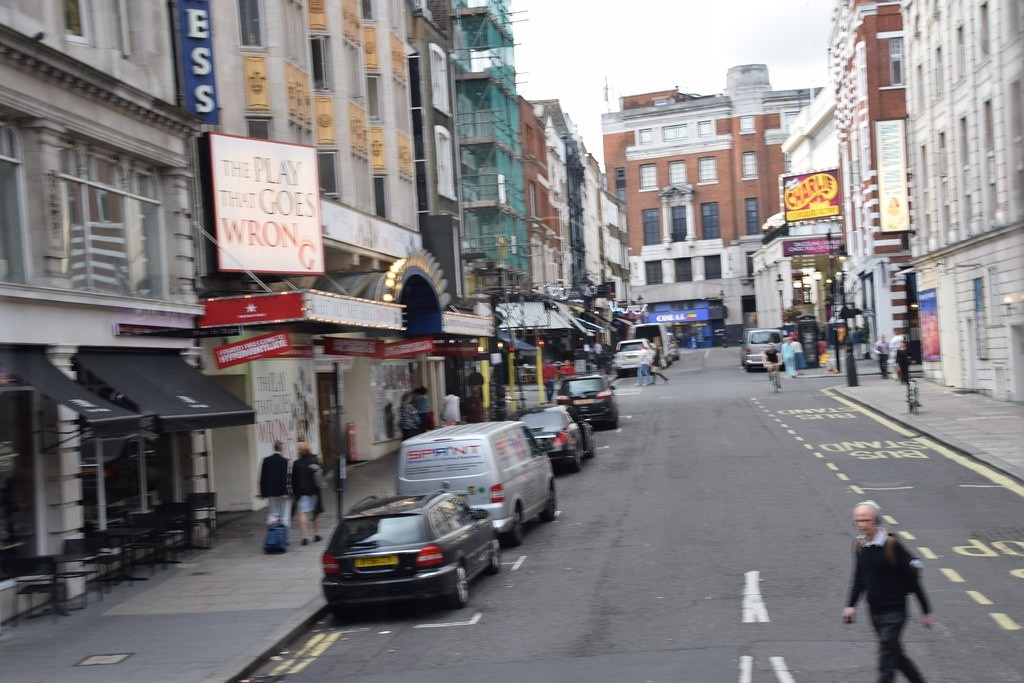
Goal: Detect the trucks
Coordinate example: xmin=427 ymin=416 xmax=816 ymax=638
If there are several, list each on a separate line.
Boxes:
xmin=629 ymin=321 xmax=675 ymax=369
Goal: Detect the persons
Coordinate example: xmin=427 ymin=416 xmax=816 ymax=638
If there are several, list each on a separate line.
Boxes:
xmin=442 ymin=390 xmax=461 ymax=425
xmin=594 ymin=339 xmax=608 ymax=364
xmin=842 ymin=504 xmax=934 ymax=683
xmin=781 ymin=338 xmax=806 ymax=377
xmin=874 ymin=334 xmax=890 ymax=379
xmin=849 ymin=326 xmax=869 ymax=358
xmin=543 ymin=359 xmax=575 ymax=400
xmin=400 ymin=388 xmax=430 ymax=441
xmin=465 ymin=388 xmax=485 ymax=423
xmin=889 ymin=332 xmax=908 ymax=384
xmin=762 ymin=343 xmax=782 ymax=389
xmin=636 ymin=345 xmax=669 ymax=386
xmin=260 ymin=442 xmax=324 ymax=545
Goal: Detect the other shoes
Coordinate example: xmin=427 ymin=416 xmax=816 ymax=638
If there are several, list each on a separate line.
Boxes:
xmin=302 ymin=538 xmax=308 ymax=545
xmin=315 ymin=535 xmax=320 ymax=541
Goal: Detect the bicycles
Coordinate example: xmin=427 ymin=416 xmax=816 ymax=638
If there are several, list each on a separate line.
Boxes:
xmin=764 ymin=364 xmax=784 ymax=394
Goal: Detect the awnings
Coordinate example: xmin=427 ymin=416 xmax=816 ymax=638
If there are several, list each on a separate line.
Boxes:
xmin=0 ymin=351 xmax=155 ymax=454
xmin=70 ymin=350 xmax=257 ymax=432
xmin=649 ymin=309 xmax=709 ymax=323
xmin=495 ymin=302 xmax=633 ymax=336
xmin=453 ymin=308 xmax=537 ymax=355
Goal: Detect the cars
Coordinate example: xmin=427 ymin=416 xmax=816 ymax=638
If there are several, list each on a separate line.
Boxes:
xmin=507 ymin=403 xmax=595 ymax=473
xmin=319 ymin=489 xmax=502 ymax=619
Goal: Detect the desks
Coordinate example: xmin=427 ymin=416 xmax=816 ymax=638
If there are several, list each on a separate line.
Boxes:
xmin=97 ymin=528 xmax=152 ymax=585
xmin=162 ymin=504 xmax=212 ymax=553
xmin=45 ymin=554 xmax=98 ymax=605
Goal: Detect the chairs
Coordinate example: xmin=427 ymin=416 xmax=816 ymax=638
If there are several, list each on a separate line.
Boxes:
xmin=9 ymin=491 xmax=216 ymax=626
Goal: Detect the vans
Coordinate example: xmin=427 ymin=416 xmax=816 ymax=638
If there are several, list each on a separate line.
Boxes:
xmin=737 ymin=327 xmax=785 ymax=372
xmin=396 ymin=417 xmax=558 ymax=550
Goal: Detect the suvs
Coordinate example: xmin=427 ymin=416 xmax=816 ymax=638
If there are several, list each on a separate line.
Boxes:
xmin=667 ymin=332 xmax=681 ymax=361
xmin=554 ymin=373 xmax=620 ymax=430
xmin=611 ymin=338 xmax=659 ymax=378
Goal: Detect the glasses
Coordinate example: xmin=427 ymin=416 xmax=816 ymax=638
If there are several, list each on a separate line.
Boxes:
xmin=854 ymin=518 xmax=878 ymax=526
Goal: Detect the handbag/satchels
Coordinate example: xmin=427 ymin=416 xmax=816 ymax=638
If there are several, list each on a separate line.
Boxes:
xmin=307 ymin=457 xmax=328 ymax=489
xmin=651 ymin=366 xmax=661 ymax=374
xmin=440 ymin=407 xmax=446 ymax=420
xmin=896 ymin=363 xmax=901 ymax=378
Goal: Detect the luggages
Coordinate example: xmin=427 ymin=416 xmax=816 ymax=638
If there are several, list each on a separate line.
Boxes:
xmin=264 ymin=500 xmax=287 ymax=553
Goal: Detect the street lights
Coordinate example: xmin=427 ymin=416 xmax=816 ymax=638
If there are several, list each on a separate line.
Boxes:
xmin=833 ymin=245 xmax=850 ymax=334
xmin=775 ymin=272 xmax=786 ymax=326
xmin=814 ymin=266 xmax=825 ymax=322
